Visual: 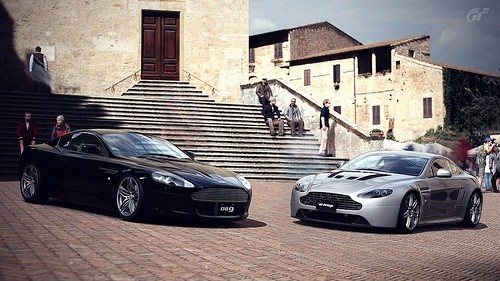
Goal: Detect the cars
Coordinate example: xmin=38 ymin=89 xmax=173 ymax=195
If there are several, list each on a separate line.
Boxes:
xmin=18 ymin=128 xmax=253 ymax=223
xmin=289 ymin=150 xmax=484 ymax=235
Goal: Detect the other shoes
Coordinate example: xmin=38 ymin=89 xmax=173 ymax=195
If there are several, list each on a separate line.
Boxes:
xmin=292 ymin=134 xmax=296 ymax=137
xmin=299 ymin=134 xmax=303 ymax=137
xmin=323 ymin=153 xmax=329 ymax=157
xmin=271 ymin=134 xmax=276 ymax=137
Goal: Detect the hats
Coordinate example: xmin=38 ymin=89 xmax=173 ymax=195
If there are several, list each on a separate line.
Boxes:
xmin=483 ymin=138 xmax=493 ymax=142
xmin=56 ymin=115 xmax=64 ymax=121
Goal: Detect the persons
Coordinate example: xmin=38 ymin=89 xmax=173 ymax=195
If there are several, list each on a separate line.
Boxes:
xmin=256 ymin=77 xmax=276 ymax=105
xmin=17 ymin=112 xmax=38 ymax=155
xmin=318 ymin=99 xmax=335 ymax=157
xmin=51 ymin=115 xmax=71 ymax=140
xmin=283 ymin=98 xmax=304 ymax=137
xmin=29 ymin=46 xmax=48 ymax=92
xmin=263 ymin=97 xmax=285 ymax=137
xmin=476 ymin=137 xmax=500 ymax=193
